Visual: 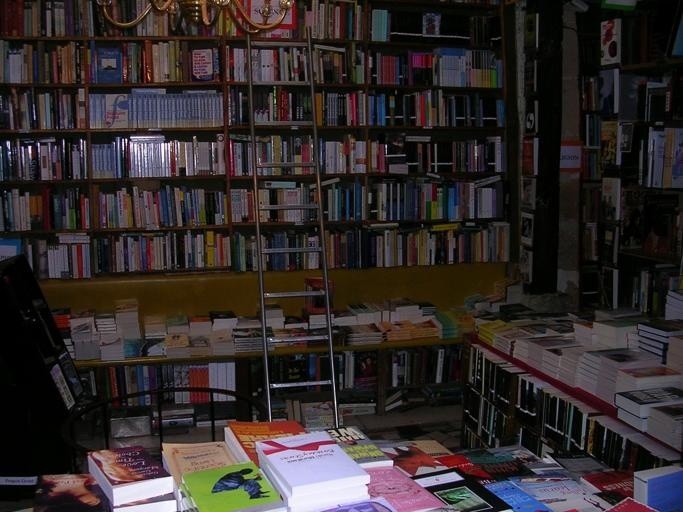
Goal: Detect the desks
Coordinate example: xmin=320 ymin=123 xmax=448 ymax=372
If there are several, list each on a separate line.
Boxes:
xmin=454 ymin=327 xmax=683 ymax=484
xmin=47 ymin=298 xmax=524 ymax=439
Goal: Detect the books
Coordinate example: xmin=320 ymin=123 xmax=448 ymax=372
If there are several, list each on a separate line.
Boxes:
xmin=1 ymin=0 xmax=683 ymax=511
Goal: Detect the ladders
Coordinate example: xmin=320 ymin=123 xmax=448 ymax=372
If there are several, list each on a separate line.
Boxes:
xmin=246 ymin=26 xmax=340 ymax=431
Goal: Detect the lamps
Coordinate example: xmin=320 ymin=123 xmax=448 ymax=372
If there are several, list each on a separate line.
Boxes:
xmin=90 ymin=0 xmax=292 ymax=40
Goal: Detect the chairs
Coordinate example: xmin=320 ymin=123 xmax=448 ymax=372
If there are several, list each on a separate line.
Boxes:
xmin=53 ymin=383 xmax=275 ymax=461
